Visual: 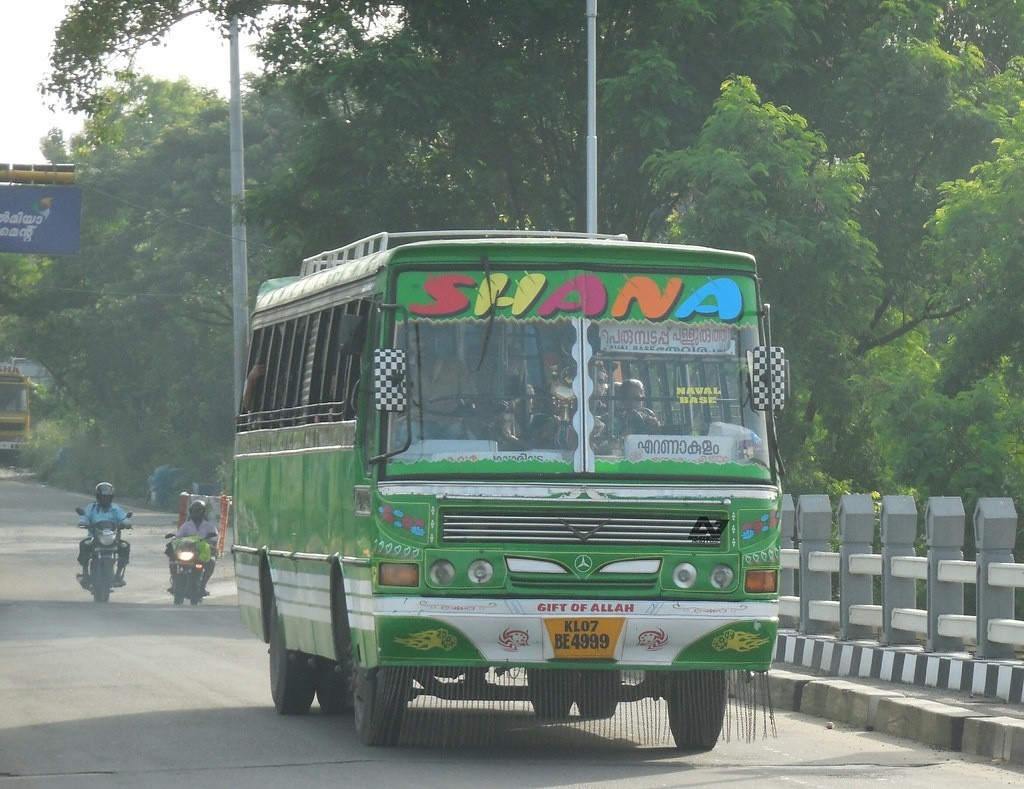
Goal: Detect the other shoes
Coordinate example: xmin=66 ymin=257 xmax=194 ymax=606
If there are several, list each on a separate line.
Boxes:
xmin=201 ymin=586 xmax=210 ymax=597
xmin=167 ymin=586 xmax=174 ymax=591
xmin=115 ymin=575 xmax=126 ymax=586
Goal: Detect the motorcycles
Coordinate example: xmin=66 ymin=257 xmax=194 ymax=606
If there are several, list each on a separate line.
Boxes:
xmin=75 ymin=507 xmax=134 ymax=602
xmin=164 ymin=531 xmax=219 ymax=605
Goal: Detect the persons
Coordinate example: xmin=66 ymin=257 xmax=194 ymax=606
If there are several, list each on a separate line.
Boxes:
xmin=164 ymin=500 xmax=218 ymax=596
xmin=77 ymin=482 xmax=132 ymax=587
xmin=242 ymin=364 xmax=266 ymax=410
xmin=403 ymin=323 xmax=479 ymax=440
xmin=483 ymin=326 xmax=609 ymax=450
xmin=605 ymin=378 xmax=661 ymax=427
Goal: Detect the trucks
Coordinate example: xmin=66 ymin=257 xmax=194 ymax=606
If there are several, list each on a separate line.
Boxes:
xmin=0 ymin=357 xmax=57 ymax=452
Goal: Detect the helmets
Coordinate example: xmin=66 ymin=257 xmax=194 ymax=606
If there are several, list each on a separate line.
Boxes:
xmin=189 ymin=500 xmax=206 ymax=519
xmin=95 ymin=482 xmax=115 ymax=507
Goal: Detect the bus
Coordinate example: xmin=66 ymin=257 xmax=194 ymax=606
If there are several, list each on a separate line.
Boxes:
xmin=232 ymin=230 xmax=790 ymax=751
